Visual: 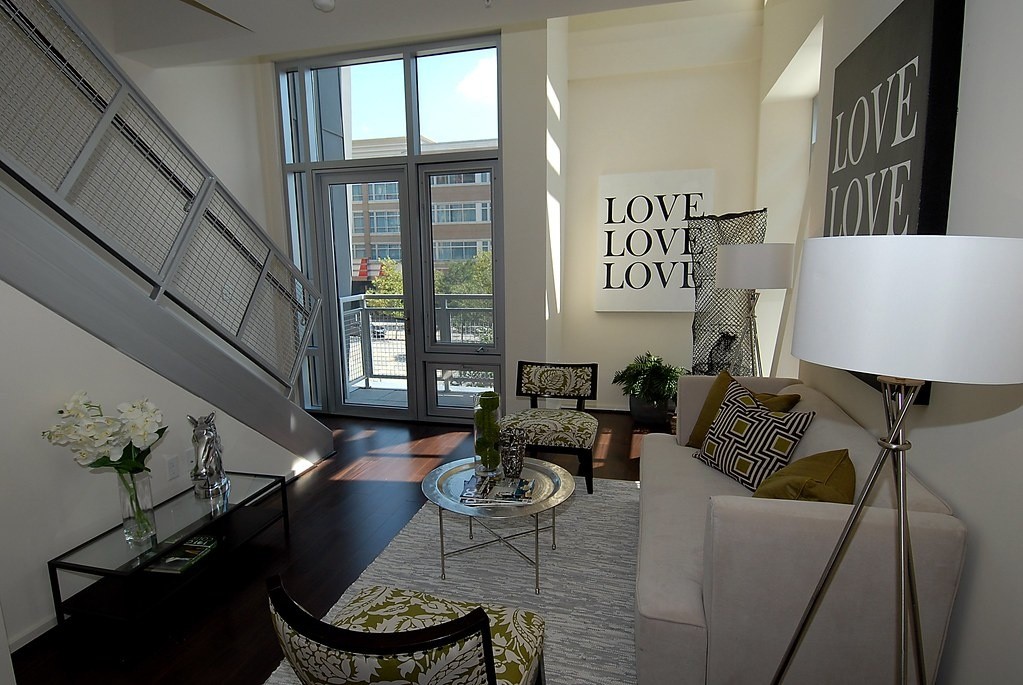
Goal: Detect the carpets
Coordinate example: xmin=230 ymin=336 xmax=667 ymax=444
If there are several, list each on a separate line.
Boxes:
xmin=263 ymin=476 xmax=640 ymax=685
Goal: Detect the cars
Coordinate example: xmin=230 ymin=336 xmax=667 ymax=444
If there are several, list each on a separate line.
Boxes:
xmin=359 ymin=321 xmax=385 ymax=338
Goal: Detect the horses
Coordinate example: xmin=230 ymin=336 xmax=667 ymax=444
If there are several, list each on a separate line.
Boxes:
xmin=186 ymin=411 xmax=231 ymax=489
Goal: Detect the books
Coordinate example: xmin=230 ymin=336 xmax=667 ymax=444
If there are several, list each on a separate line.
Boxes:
xmin=144 ymin=535 xmax=225 ymax=573
xmin=458 ymin=474 xmax=535 ymax=508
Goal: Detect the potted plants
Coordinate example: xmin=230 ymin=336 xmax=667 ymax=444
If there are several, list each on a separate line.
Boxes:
xmin=610 ymin=350 xmax=691 ymax=428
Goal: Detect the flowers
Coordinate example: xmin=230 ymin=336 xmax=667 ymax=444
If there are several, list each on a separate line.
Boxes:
xmin=40 ymin=389 xmax=169 ymax=474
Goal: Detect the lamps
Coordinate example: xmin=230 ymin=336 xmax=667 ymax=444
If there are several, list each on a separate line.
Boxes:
xmin=716 ymin=243 xmax=794 ymax=376
xmin=768 ymin=236 xmax=1023 ymax=685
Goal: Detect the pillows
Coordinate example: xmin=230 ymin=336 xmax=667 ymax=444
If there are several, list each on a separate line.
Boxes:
xmin=751 ymin=449 xmax=855 ymax=505
xmin=686 ymin=375 xmax=801 ymax=448
xmin=692 ymin=381 xmax=817 ymax=492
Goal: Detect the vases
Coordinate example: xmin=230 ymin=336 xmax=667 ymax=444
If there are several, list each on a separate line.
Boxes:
xmin=117 ymin=470 xmax=157 ymax=541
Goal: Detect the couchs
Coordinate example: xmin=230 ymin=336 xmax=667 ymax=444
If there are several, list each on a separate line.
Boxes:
xmin=636 ymin=376 xmax=965 ymax=685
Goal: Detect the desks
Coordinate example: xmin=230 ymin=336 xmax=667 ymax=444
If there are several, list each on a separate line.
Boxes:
xmin=421 ymin=457 xmax=575 ymax=595
xmin=46 ymin=470 xmax=290 ymax=651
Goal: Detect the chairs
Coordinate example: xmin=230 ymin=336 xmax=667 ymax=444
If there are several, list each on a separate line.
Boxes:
xmin=499 ymin=360 xmax=599 ymax=494
xmin=267 ymin=578 xmax=546 ymax=685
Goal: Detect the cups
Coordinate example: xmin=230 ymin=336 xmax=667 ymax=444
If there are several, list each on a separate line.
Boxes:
xmin=473 ymin=392 xmax=501 ymax=476
